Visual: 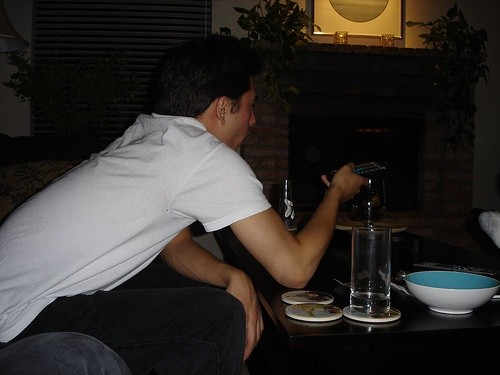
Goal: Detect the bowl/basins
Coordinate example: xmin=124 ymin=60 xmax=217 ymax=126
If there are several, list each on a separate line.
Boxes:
xmin=403 ymin=271 xmax=500 ymax=314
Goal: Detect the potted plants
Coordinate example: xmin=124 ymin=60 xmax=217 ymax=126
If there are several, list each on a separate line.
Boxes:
xmin=402 ymin=2 xmax=490 ymax=152
xmin=220 ymin=0 xmax=321 ymax=116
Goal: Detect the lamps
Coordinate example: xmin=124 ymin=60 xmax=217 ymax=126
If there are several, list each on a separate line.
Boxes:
xmin=0 ymin=0 xmax=30 ymax=52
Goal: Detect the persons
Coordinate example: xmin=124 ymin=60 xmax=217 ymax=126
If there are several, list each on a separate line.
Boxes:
xmin=0 ymin=34 xmax=370 ymax=375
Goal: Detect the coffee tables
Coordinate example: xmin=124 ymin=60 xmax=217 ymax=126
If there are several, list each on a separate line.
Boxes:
xmin=217 ymin=227 xmax=499 ymax=375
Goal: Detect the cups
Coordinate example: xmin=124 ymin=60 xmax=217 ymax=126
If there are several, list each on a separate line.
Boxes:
xmin=351 ymin=179 xmax=387 ymax=222
xmin=351 ymin=226 xmax=392 ymax=318
xmin=381 ymin=34 xmax=395 ymax=46
xmin=333 ymin=31 xmax=347 ymax=44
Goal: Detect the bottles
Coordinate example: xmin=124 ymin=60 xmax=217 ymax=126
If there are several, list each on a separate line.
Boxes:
xmin=278 ymin=178 xmax=297 ymax=236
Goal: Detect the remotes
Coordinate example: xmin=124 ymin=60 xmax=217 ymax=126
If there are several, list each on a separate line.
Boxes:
xmin=411 ymin=261 xmax=500 ymax=278
xmin=326 ymin=161 xmax=387 ymax=183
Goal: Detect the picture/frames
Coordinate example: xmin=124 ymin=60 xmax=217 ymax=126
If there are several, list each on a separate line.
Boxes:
xmin=306 ymin=0 xmax=406 ymax=40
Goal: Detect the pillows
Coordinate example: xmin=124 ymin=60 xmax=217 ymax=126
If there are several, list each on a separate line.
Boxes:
xmin=0 ymin=160 xmax=81 ymax=223
